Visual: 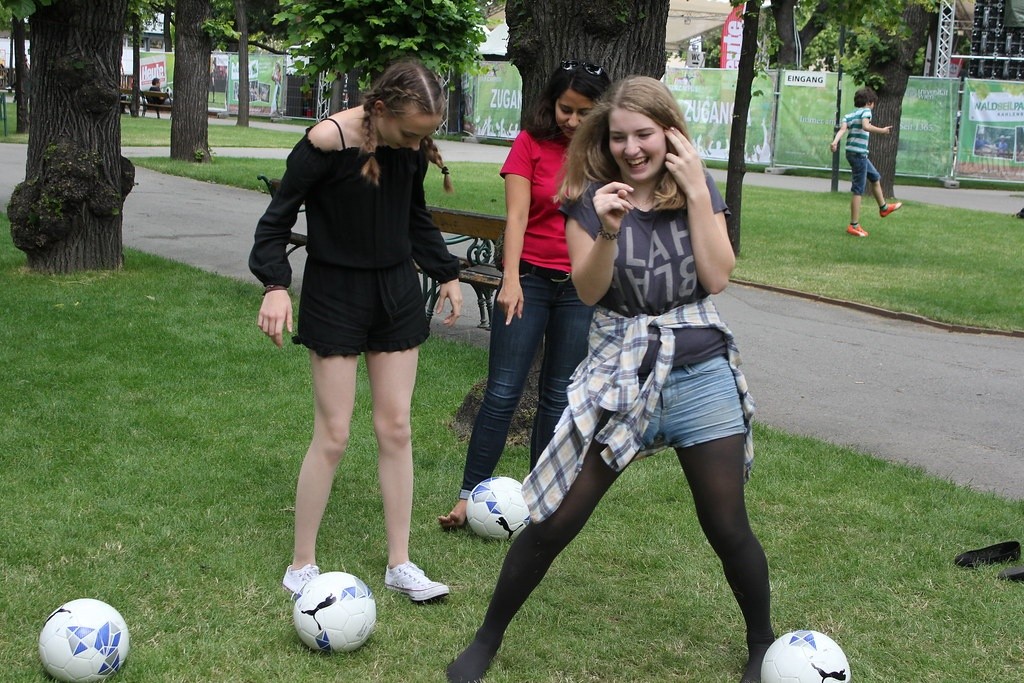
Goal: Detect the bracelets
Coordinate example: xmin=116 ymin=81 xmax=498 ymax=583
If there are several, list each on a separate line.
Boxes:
xmin=264 ymin=284 xmax=287 ymax=292
xmin=597 ymin=224 xmax=622 ymax=241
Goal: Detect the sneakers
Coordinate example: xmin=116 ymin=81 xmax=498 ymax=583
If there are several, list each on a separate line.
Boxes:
xmin=847 ymin=224 xmax=868 ymax=237
xmin=384 ymin=561 xmax=449 ymax=601
xmin=880 ymin=202 xmax=902 ymax=217
xmin=282 ymin=564 xmax=320 ymax=600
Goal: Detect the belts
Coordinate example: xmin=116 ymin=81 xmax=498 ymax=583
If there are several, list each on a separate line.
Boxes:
xmin=519 ymin=260 xmax=572 ymax=282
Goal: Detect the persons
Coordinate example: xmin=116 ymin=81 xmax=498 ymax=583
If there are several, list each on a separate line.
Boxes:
xmin=434 ymin=59 xmax=611 ymax=528
xmin=271 ymin=62 xmax=282 ymax=114
xmin=443 ymin=75 xmax=779 ymax=683
xmin=147 ymin=78 xmax=175 ymax=104
xmin=829 ymin=88 xmax=904 ymax=238
xmin=247 ymin=59 xmax=463 ymax=597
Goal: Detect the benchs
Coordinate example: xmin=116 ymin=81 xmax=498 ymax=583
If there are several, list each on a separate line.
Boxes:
xmin=259 ymin=174 xmax=507 ymax=331
xmin=120 ymin=87 xmax=172 ymax=119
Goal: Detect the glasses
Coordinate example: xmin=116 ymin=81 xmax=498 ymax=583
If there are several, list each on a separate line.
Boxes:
xmin=561 ymin=60 xmax=607 ymax=81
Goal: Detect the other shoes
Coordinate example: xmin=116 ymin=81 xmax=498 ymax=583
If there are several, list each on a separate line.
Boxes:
xmin=955 ymin=541 xmax=1021 ymax=567
xmin=998 ymin=567 xmax=1024 ymax=581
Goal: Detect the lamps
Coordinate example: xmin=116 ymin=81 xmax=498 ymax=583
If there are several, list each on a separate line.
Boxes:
xmin=684 ymin=18 xmax=691 ymax=25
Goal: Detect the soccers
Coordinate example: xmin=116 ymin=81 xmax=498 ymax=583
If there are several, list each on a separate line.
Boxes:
xmin=38 ymin=598 xmax=130 ymax=683
xmin=465 ymin=476 xmax=531 ymax=540
xmin=760 ymin=629 xmax=852 ymax=683
xmin=292 ymin=572 xmax=376 ymax=653
